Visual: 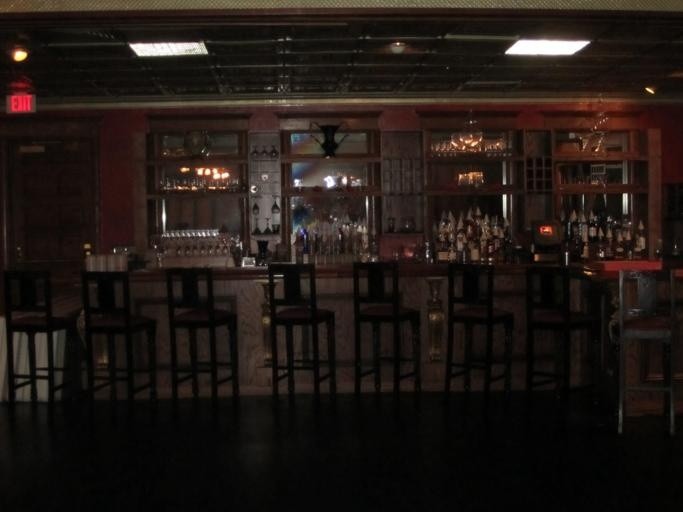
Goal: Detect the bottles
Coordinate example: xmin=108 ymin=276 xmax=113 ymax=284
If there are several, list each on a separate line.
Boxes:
xmin=437 ymin=208 xmax=647 ymax=260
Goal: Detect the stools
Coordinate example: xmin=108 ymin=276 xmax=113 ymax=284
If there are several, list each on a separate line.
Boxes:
xmin=446 ymin=263 xmax=515 ymax=418
xmin=524 ymin=265 xmax=601 ymax=425
xmin=350 ymin=263 xmax=423 ymax=423
xmin=265 ymin=262 xmax=339 ymax=429
xmin=79 ymin=267 xmax=164 ymax=435
xmin=1 ymin=264 xmax=86 ymax=435
xmin=158 ymin=266 xmax=243 ymax=435
xmin=615 ymin=268 xmax=677 ymax=435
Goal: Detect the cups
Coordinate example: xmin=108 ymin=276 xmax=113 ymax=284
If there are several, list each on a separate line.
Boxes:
xmin=272 ymin=224 xmax=280 ymax=233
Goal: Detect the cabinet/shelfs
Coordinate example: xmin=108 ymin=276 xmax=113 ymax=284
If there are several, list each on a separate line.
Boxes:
xmin=1 ymin=109 xmax=648 ymax=262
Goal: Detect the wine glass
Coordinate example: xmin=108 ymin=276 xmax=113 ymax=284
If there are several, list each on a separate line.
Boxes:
xmin=252 ymin=218 xmax=262 ymax=235
xmin=251 ymin=194 xmax=262 ymax=215
xmin=263 ymin=217 xmax=272 ymax=234
xmin=271 ymin=194 xmax=280 ymax=215
